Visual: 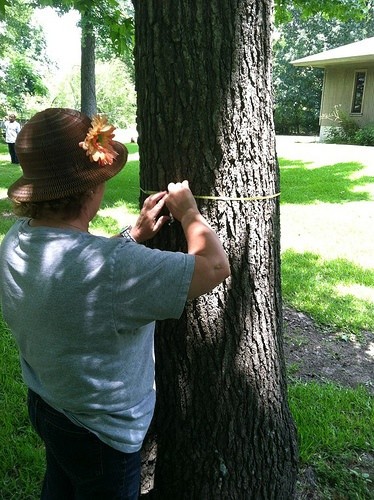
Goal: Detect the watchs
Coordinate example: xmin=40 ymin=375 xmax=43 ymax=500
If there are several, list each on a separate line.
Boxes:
xmin=120 ymin=224 xmax=137 ymax=242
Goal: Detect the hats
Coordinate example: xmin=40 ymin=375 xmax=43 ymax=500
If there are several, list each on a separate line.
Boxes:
xmin=7 ymin=108 xmax=129 ymax=204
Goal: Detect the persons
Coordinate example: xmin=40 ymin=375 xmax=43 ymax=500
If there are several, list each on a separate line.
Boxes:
xmin=0 ymin=108 xmax=231 ymax=500
xmin=4 ymin=113 xmax=22 ymax=165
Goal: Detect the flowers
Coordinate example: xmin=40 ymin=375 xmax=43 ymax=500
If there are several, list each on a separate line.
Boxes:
xmin=79 ymin=113 xmax=119 ymax=168
